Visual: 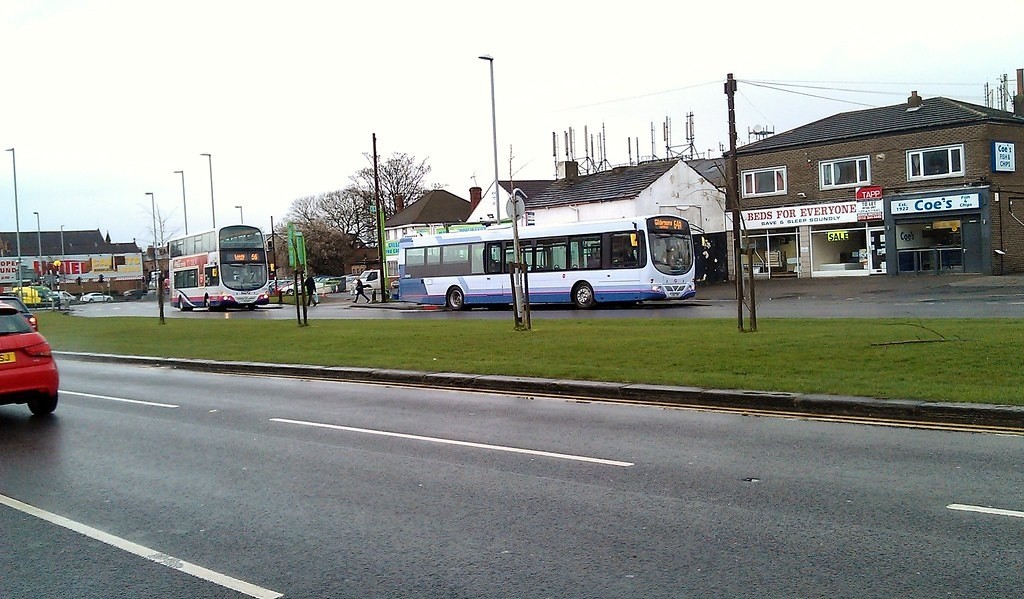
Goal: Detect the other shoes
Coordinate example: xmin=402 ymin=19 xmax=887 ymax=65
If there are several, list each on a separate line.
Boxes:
xmin=352 ymin=300 xmax=357 ymax=303
xmin=367 ymin=299 xmax=370 ymax=303
xmin=313 ymin=303 xmax=317 ymax=306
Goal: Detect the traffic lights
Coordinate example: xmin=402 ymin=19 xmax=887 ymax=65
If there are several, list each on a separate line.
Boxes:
xmin=148 ymin=272 xmax=151 ymax=281
xmin=100 ymin=275 xmax=104 ymax=283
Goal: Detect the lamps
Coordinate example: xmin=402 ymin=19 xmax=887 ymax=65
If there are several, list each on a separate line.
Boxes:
xmin=796 ymin=192 xmax=807 ymax=198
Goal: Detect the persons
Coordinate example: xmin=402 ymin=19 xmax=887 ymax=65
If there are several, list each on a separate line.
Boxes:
xmin=700 ymin=249 xmax=718 ymax=281
xmin=352 ymin=276 xmax=370 ymax=303
xmin=304 ymin=273 xmax=316 ymax=307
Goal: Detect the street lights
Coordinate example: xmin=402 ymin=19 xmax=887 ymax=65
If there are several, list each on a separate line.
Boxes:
xmin=479 ymin=54 xmax=500 ymax=223
xmin=145 ymin=193 xmax=159 ymax=290
xmin=200 ymin=154 xmax=215 ymax=229
xmin=174 ymin=170 xmax=187 ymax=234
xmin=60 ymin=225 xmax=66 ymax=290
xmin=34 ymin=212 xmax=45 ymax=286
xmin=5 ymin=148 xmax=22 ymax=287
xmin=235 ymin=206 xmax=243 ymax=224
xmin=54 ymin=260 xmax=61 ymax=310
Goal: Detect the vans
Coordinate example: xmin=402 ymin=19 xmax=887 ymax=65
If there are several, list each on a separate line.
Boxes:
xmin=352 ymin=270 xmax=381 ymax=290
xmin=13 ymin=286 xmax=60 ymax=306
xmin=54 ymin=292 xmax=77 ymax=302
xmin=163 ymin=279 xmax=170 ymax=296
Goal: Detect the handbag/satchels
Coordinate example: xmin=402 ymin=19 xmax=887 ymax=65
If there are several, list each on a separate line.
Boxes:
xmin=314 ymin=292 xmax=319 ymax=303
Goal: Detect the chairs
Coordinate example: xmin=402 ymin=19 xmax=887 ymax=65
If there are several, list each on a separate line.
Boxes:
xmin=765 ymin=250 xmax=783 ymax=272
xmin=492 ymin=259 xmax=580 ymax=270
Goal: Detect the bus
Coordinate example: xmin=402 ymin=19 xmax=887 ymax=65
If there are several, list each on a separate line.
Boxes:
xmin=168 ymin=226 xmax=274 ymax=311
xmin=398 ymin=224 xmax=706 ymax=313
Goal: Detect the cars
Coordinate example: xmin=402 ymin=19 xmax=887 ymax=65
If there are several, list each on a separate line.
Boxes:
xmin=268 ymin=275 xmax=358 ymax=296
xmin=0 ymin=297 xmax=38 ymax=332
xmin=124 ymin=289 xmax=147 ymax=300
xmin=0 ymin=302 xmax=59 ymax=416
xmin=80 ymin=293 xmax=114 ymax=302
